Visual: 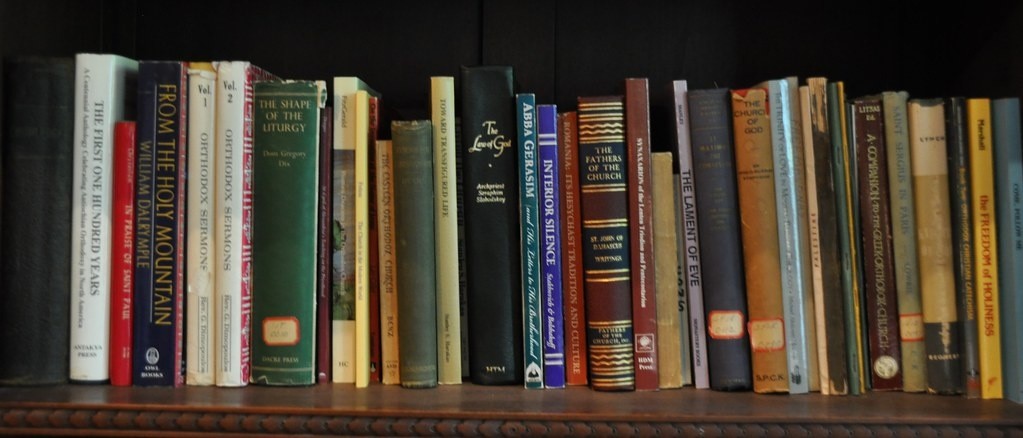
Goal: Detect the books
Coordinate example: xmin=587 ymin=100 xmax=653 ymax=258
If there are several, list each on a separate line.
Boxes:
xmin=0 ymin=54 xmax=74 ymax=385
xmin=69 ymin=53 xmax=1023 ymax=405
